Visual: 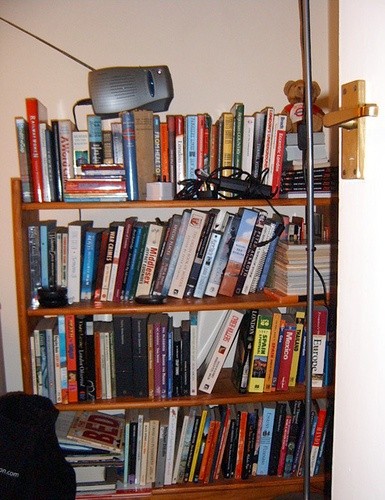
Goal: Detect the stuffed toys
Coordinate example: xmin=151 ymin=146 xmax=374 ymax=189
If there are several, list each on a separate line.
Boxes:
xmin=282 ymin=79 xmax=324 ymax=132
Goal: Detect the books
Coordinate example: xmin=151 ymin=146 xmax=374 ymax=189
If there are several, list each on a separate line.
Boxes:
xmin=280 ymin=132 xmax=339 ymax=202
xmin=13 ymin=98 xmax=286 ymax=205
xmin=26 ymin=206 xmax=331 ymax=307
xmin=52 ymin=400 xmax=333 ymax=497
xmin=26 ymin=309 xmax=334 ymax=399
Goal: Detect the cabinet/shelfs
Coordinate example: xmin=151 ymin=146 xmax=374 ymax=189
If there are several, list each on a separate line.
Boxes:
xmin=9 ymin=177 xmax=338 ymax=499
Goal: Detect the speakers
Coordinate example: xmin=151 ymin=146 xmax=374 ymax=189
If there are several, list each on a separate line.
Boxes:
xmin=88 ymin=65 xmax=174 ymax=119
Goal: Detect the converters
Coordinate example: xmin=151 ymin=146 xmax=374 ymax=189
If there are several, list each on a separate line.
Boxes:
xmin=218 ymin=175 xmax=273 ymax=199
